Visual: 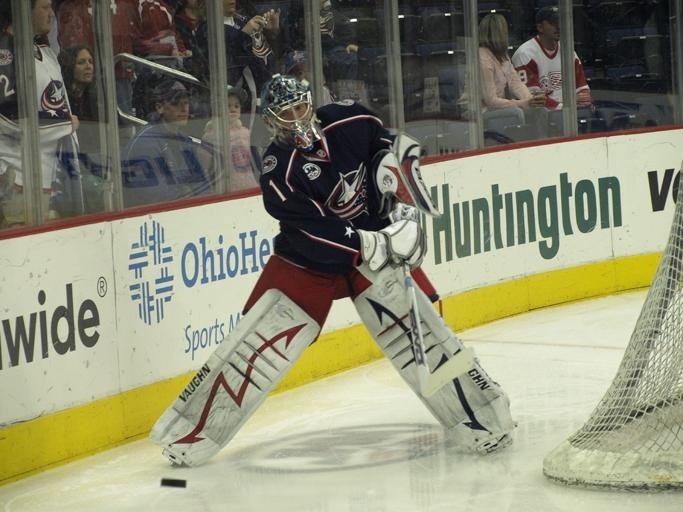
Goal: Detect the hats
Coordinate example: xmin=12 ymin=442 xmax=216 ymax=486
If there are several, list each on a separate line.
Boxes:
xmin=207 ymin=85 xmax=248 ymax=114
xmin=151 ymin=79 xmax=197 ymax=101
xmin=285 ymin=50 xmax=308 ymax=73
xmin=535 ymin=6 xmax=558 ymax=22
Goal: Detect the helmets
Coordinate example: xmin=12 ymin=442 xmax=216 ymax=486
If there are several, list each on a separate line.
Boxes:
xmin=260 ymin=74 xmax=323 ymax=153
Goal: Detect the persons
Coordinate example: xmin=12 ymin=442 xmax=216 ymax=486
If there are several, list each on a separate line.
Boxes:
xmin=509 ymin=8 xmax=654 ymax=132
xmin=477 ymin=13 xmax=549 ymax=142
xmin=150 ymin=72 xmax=521 ymax=466
xmin=1 ymin=0 xmax=377 ymax=227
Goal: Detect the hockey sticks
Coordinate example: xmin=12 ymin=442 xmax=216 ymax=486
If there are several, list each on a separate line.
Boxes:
xmin=403 ymin=262 xmax=475 ymax=396
